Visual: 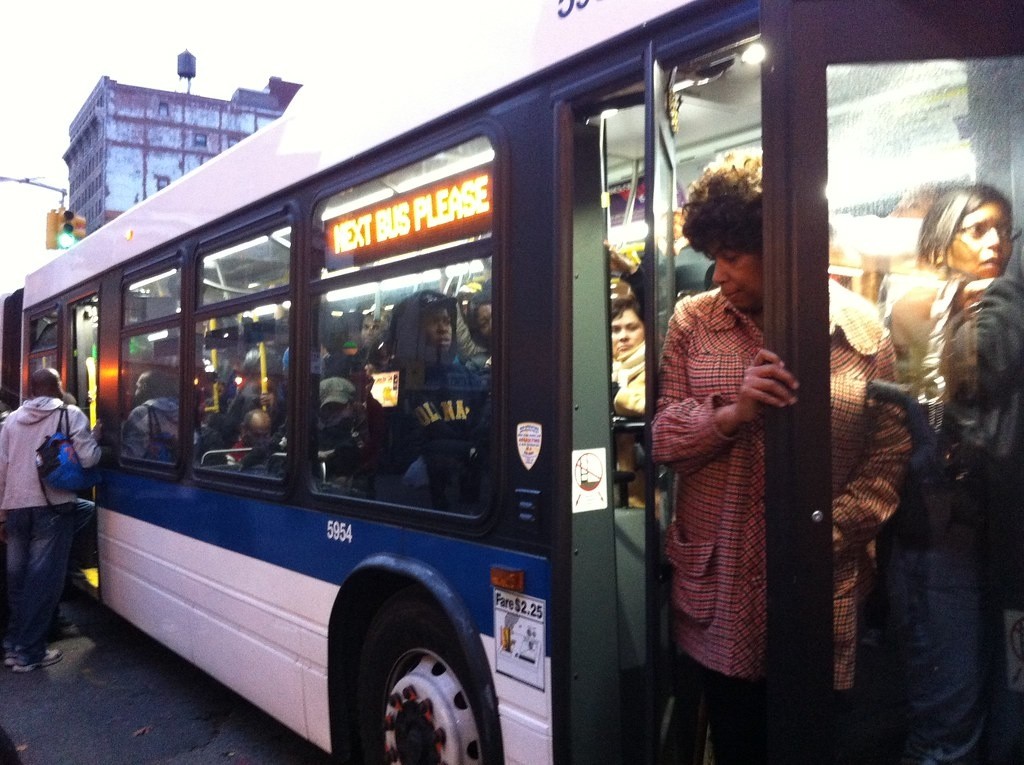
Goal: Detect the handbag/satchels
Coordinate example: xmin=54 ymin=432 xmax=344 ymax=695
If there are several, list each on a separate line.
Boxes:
xmin=135 ymin=407 xmax=178 ymax=463
xmin=34 ymin=404 xmax=101 ymax=490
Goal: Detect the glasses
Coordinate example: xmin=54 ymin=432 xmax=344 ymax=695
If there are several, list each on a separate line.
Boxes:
xmin=958 ymin=221 xmax=1024 ymax=243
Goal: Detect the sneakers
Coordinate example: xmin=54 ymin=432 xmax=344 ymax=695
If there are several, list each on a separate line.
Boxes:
xmin=4 ymin=646 xmax=64 ymax=673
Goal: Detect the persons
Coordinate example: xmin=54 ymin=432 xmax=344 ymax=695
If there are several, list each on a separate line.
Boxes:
xmin=829 ymin=179 xmax=1023 ymax=765
xmin=122 ymin=201 xmax=713 ymax=511
xmin=651 ymin=151 xmax=914 ymax=765
xmin=1 ymin=368 xmax=103 ymax=673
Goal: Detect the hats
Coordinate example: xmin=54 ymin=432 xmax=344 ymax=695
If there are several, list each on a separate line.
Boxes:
xmin=319 ymin=378 xmax=355 ymax=411
xmin=419 ymin=292 xmax=456 ymax=318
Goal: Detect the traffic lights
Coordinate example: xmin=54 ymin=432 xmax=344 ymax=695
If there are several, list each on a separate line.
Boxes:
xmin=73 ymin=214 xmax=86 ymax=239
xmin=58 ymin=210 xmax=76 ymax=249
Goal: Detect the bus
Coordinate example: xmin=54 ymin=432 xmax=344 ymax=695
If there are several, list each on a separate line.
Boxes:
xmin=1 ymin=1 xmax=1024 ymax=765
